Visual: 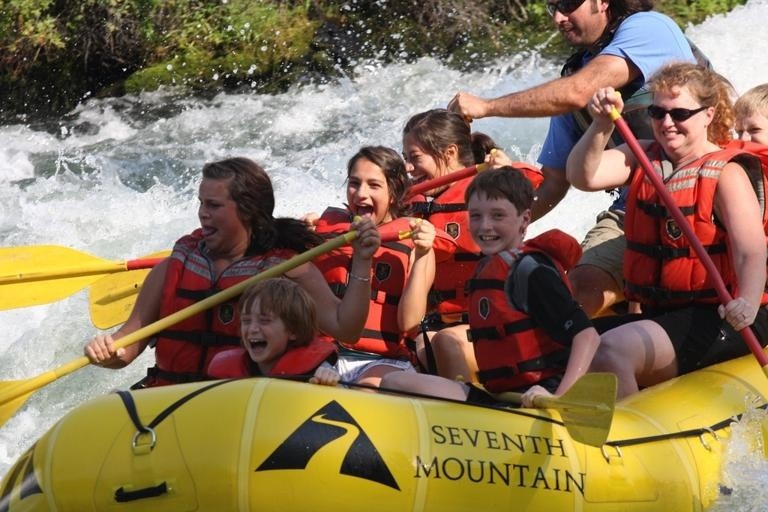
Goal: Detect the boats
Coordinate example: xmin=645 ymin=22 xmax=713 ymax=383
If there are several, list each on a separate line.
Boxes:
xmin=0 ymin=346 xmax=767 ymax=512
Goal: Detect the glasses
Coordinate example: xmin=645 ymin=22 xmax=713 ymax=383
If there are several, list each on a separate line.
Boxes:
xmin=544 ymin=0 xmax=586 ymax=18
xmin=647 ymin=104 xmax=709 ymax=122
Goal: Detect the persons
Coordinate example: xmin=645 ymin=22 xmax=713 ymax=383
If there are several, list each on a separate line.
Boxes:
xmin=401 ymin=108 xmax=544 ymax=311
xmin=731 ymin=82 xmax=768 ymax=149
xmin=565 ymin=61 xmax=768 ymax=400
xmin=208 ymin=278 xmax=340 ymax=385
xmin=380 ymin=165 xmax=601 ymax=406
xmin=448 ymin=0 xmax=703 ymax=224
xmin=468 ymin=131 xmax=583 ymax=266
xmin=309 ymin=143 xmax=438 ymax=388
xmin=84 ymin=157 xmax=382 ymax=388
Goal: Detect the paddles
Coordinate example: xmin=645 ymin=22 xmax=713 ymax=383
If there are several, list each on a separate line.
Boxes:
xmin=88 ymin=166 xmax=485 ymax=329
xmin=0 ymin=218 xmax=412 ymax=309
xmin=0 ymin=221 xmax=359 ymax=431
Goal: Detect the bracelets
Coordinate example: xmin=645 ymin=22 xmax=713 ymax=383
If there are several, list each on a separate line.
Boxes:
xmin=351 ymin=272 xmax=372 ymax=284
